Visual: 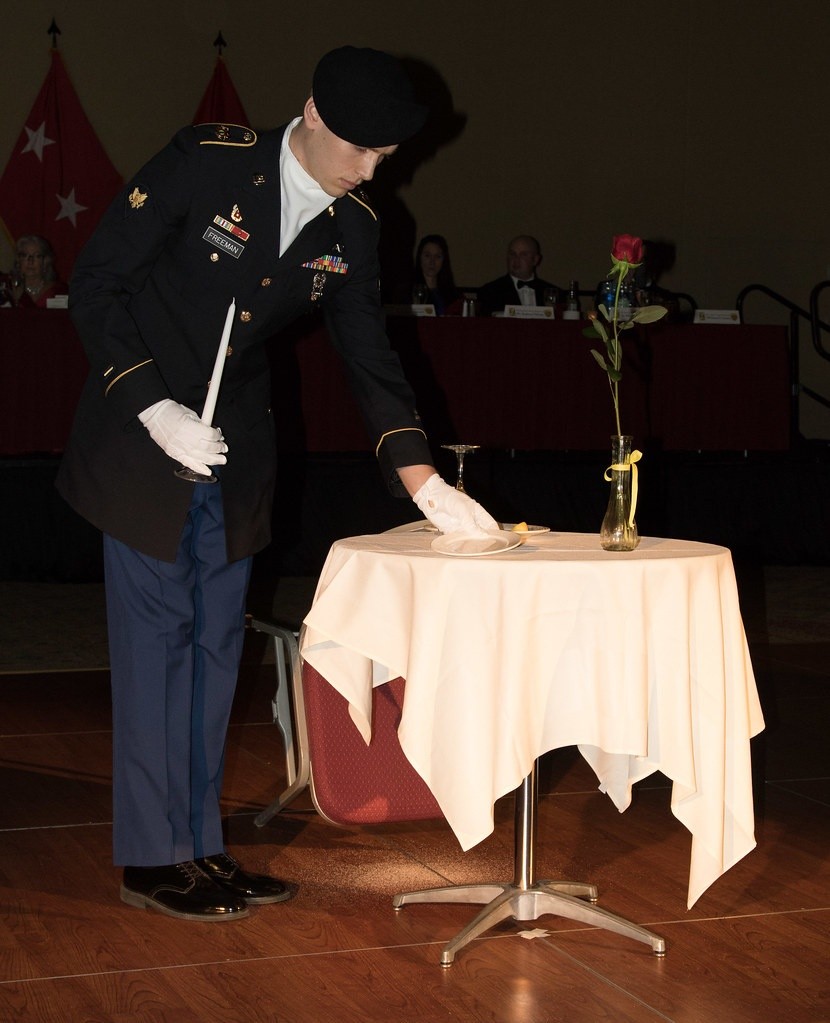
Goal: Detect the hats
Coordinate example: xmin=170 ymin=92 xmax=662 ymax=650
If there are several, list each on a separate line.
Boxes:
xmin=311 ymin=45 xmax=427 ymax=148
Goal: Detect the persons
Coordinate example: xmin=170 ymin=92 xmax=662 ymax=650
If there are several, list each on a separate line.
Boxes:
xmin=50 ymin=44 xmax=507 ymax=926
xmin=473 ymin=234 xmax=570 ymax=310
xmin=405 ymin=234 xmax=467 ymax=310
xmin=0 ymin=234 xmax=70 ymax=309
xmin=596 ymin=234 xmax=681 ymax=319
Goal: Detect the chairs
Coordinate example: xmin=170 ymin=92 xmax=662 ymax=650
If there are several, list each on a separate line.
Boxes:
xmin=247 ymin=619 xmax=446 ymax=830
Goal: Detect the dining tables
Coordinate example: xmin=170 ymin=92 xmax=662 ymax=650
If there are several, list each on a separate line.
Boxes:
xmin=333 ymin=531 xmax=732 ymax=968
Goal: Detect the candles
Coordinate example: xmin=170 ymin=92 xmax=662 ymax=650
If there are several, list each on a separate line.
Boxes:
xmin=200 ymin=296 xmax=236 ymax=427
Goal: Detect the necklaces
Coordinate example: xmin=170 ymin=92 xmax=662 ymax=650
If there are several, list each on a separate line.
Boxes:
xmin=24 ymin=271 xmax=47 ymax=296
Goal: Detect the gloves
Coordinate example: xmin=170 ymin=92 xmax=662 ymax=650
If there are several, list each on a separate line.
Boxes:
xmin=138 ymin=398 xmax=228 ymax=475
xmin=413 ymin=472 xmax=500 ymax=541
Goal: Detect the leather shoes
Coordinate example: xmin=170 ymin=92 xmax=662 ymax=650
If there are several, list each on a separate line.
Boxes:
xmin=120 ymin=853 xmax=294 ymax=923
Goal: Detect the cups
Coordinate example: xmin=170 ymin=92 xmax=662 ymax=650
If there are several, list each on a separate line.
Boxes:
xmin=412 ymin=283 xmax=428 ymax=306
xmin=461 ymin=297 xmax=479 ymax=318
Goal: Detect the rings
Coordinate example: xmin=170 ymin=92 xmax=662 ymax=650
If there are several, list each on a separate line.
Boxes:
xmin=14 ymin=281 xmax=19 ymax=286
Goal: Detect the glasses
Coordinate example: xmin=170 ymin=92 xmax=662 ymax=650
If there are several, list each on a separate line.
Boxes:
xmin=18 ymin=252 xmax=44 ymax=260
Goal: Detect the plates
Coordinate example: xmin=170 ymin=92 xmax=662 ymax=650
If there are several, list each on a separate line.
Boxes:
xmin=431 ymin=529 xmax=523 ymax=556
xmin=424 ymin=524 xmax=439 ymax=532
xmin=501 ymin=523 xmax=551 ymax=537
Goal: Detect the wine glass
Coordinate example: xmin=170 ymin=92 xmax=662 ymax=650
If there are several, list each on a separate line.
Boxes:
xmin=544 ymin=288 xmax=559 ymax=305
xmin=441 ymin=445 xmax=481 ymax=494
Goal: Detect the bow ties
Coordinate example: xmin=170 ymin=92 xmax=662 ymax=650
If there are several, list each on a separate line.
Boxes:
xmin=516 ymin=279 xmax=536 ymax=289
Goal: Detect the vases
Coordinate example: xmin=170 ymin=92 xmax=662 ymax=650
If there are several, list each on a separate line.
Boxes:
xmin=598 ymin=436 xmax=639 ymax=551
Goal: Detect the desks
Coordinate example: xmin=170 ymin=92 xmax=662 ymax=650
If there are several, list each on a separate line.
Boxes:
xmin=1 ymin=308 xmax=793 ymax=459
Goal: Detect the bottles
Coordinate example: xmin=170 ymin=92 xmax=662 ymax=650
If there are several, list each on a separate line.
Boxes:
xmin=567 ymin=280 xmax=579 ymax=311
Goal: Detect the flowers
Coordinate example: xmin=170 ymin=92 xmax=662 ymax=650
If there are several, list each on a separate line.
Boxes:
xmin=587 ymin=232 xmax=670 ymax=543
